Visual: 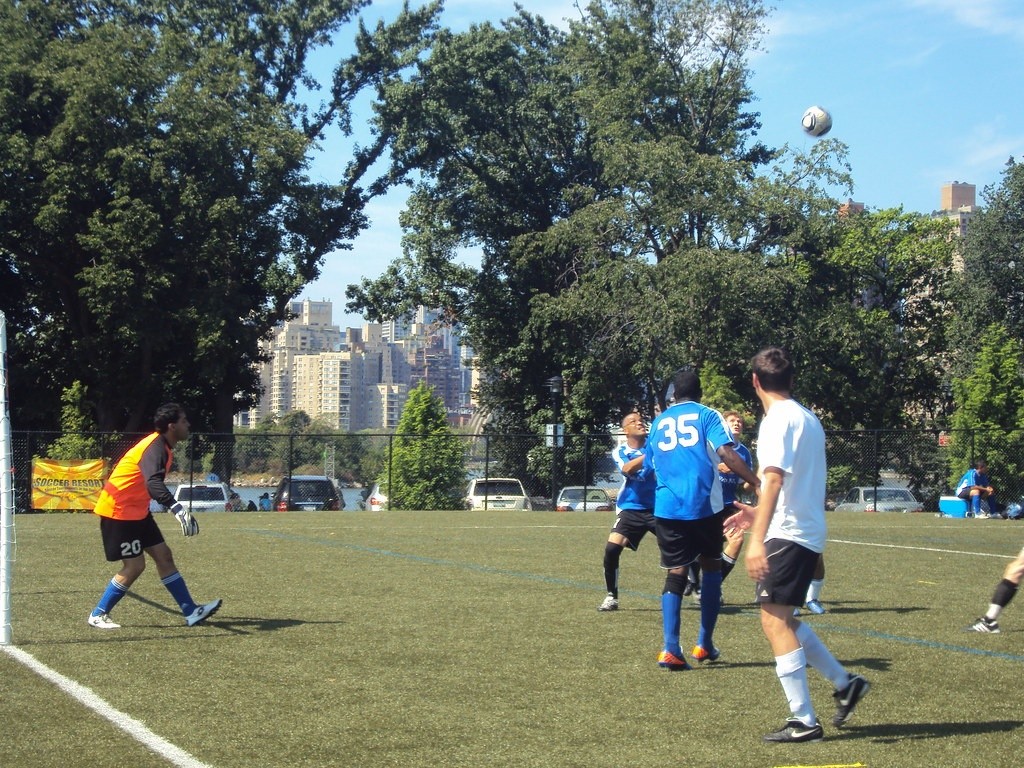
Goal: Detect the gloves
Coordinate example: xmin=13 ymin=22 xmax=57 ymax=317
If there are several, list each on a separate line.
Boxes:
xmin=168 ymin=502 xmax=200 ymax=537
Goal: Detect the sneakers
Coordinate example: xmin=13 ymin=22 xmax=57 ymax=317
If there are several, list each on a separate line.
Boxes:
xmin=597 ymin=592 xmax=619 ymax=611
xmin=184 ymin=599 xmax=223 ymax=626
xmin=960 ymin=616 xmax=1000 ymax=633
xmin=806 ymin=598 xmax=825 ymax=614
xmin=691 ymin=645 xmax=719 ymax=661
xmin=764 ymin=715 xmax=823 ymax=743
xmin=683 ymin=574 xmax=699 ymax=596
xmin=987 ymin=512 xmax=1003 ymax=519
xmin=88 ymin=612 xmax=121 ymax=629
xmin=695 ymin=589 xmax=702 ymax=602
xmin=976 ymin=512 xmax=989 ymax=519
xmin=794 ymin=608 xmax=800 ymax=616
xmin=832 ymin=672 xmax=870 ymax=730
xmin=658 ymin=646 xmax=692 ymax=670
xmin=718 ymin=583 xmax=724 ymax=607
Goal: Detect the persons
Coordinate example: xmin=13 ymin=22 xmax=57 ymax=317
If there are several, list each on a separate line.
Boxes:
xmin=226 ymin=489 xmax=257 ymax=512
xmin=629 ymin=369 xmax=762 ymax=669
xmin=744 ymin=464 xmax=826 ymax=616
xmin=722 ymin=347 xmax=870 ymax=741
xmin=598 ymin=413 xmax=702 ymax=610
xmin=88 ymin=403 xmax=222 ymax=628
xmin=957 ymin=459 xmax=1002 ymax=519
xmin=682 ymin=408 xmax=754 ymax=603
xmin=962 ymin=547 xmax=1024 ymax=633
xmin=259 ymin=492 xmax=271 ymax=511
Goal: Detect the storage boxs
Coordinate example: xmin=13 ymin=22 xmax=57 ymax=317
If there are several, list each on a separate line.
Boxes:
xmin=939 ymin=496 xmax=971 ymax=518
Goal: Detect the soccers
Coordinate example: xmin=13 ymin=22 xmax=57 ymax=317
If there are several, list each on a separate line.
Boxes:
xmin=800 ymin=105 xmax=832 ymax=138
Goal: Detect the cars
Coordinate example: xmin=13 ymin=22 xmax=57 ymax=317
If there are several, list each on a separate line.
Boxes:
xmin=557 ymin=487 xmax=614 ymax=510
xmin=361 ymin=481 xmax=390 ymax=512
xmin=465 ymin=476 xmax=532 ymax=510
xmin=271 ymin=474 xmax=343 ymax=512
xmin=174 ymin=482 xmax=235 ymax=514
xmin=832 ymin=485 xmax=924 ymax=514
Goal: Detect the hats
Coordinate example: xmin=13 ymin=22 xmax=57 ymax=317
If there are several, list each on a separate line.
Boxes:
xmin=664 ymin=384 xmax=676 ymax=402
